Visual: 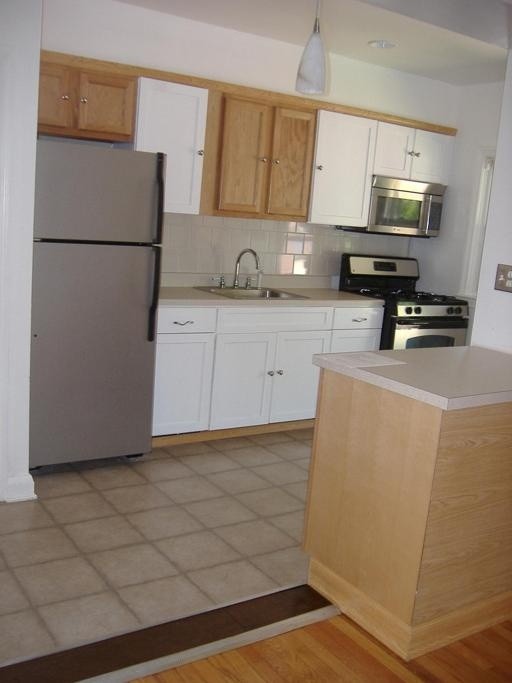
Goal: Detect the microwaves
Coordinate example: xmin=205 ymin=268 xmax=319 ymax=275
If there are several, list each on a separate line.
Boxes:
xmin=334 ymin=176 xmax=448 ymax=238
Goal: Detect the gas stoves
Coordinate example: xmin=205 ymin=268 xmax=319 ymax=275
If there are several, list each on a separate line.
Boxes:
xmin=360 ymin=286 xmax=469 ymax=317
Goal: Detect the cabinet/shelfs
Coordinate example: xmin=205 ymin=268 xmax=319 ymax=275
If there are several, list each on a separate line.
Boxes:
xmin=37 ymin=48 xmax=139 ymax=143
xmin=334 ymin=304 xmax=385 ymax=353
xmin=149 ymin=305 xmax=216 ymax=447
xmin=216 ymin=87 xmax=317 ymax=223
xmin=209 ymin=305 xmax=334 ymax=448
xmin=309 ymin=97 xmax=378 ymax=230
xmin=118 ymin=66 xmax=210 ymax=214
xmin=374 ymin=111 xmax=458 ymax=186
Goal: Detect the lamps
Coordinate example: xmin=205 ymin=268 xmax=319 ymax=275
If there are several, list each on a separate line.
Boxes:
xmin=292 ymin=2 xmax=328 ymax=97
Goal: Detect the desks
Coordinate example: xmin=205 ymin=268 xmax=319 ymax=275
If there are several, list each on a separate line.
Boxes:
xmin=298 ymin=345 xmax=511 ymax=664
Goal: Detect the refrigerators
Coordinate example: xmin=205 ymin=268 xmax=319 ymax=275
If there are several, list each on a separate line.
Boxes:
xmin=29 ymin=138 xmax=168 ymax=472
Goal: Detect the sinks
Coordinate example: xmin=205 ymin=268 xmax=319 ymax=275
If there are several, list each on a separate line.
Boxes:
xmin=192 ymin=286 xmax=310 ymax=301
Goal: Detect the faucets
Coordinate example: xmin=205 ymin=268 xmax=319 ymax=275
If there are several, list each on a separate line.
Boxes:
xmin=233 ymin=248 xmax=261 ymax=288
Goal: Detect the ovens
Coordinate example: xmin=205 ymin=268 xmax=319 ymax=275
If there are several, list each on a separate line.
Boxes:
xmin=380 ymin=317 xmax=468 ymax=352
